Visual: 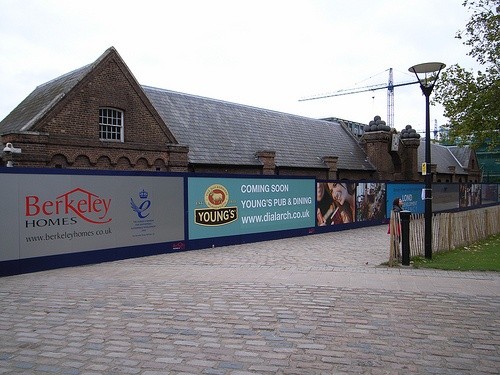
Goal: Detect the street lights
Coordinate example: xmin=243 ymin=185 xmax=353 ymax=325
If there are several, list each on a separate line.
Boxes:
xmin=408 ymin=61 xmax=442 ymax=261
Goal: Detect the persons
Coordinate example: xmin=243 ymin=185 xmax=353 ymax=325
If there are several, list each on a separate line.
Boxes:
xmin=317 ymin=183 xmax=355 ymax=226
xmin=392 ymin=198 xmax=404 ymax=258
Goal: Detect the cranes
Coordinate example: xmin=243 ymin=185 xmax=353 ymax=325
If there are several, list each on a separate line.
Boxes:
xmin=297 ymin=68 xmax=440 ymax=130
